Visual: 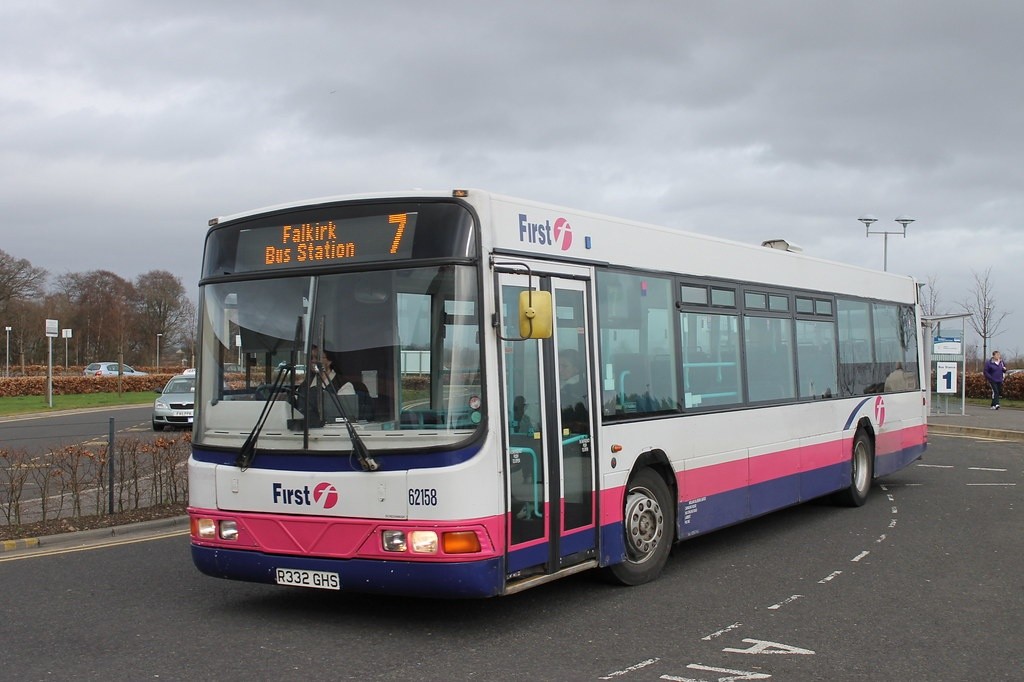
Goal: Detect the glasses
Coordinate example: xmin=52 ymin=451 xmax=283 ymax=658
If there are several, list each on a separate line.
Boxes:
xmin=311 ymin=354 xmax=328 ymax=361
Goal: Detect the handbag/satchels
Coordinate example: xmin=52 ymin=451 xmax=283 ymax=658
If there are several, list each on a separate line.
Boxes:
xmin=1001 ymin=362 xmax=1006 ymax=378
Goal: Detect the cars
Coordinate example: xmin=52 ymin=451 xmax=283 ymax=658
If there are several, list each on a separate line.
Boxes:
xmin=83 ymin=362 xmax=150 ymax=377
xmin=274 ymin=362 xmax=320 ymax=375
xmin=151 ymin=368 xmax=235 ymax=432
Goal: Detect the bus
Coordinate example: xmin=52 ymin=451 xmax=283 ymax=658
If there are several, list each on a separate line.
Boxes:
xmin=185 ymin=188 xmax=932 ymax=603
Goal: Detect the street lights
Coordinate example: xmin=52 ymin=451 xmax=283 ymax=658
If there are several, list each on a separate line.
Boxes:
xmin=156 ymin=333 xmax=164 ymax=374
xmin=5 ymin=327 xmax=12 ymax=377
xmin=986 ymin=306 xmax=992 ymax=357
xmin=857 ymin=212 xmax=916 ymax=272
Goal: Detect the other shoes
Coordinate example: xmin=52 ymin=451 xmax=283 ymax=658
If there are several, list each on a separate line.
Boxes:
xmin=996 ymin=404 xmax=1000 ymax=410
xmin=990 ymin=406 xmax=996 ymax=409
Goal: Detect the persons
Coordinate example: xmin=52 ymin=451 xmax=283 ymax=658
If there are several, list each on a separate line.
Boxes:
xmin=516 ymin=348 xmax=590 ymax=520
xmin=293 ymin=340 xmax=359 ymax=420
xmin=983 ymin=351 xmax=1007 ymax=409
